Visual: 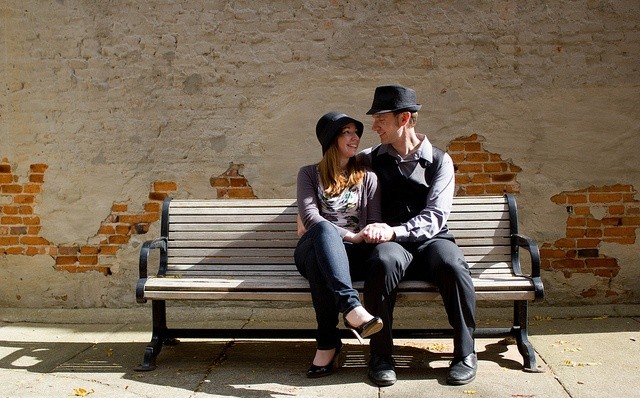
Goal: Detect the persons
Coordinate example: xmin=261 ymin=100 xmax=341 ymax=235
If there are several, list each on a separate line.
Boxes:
xmin=294 ymin=110 xmax=390 ymax=378
xmin=298 ymin=85 xmax=478 ymax=385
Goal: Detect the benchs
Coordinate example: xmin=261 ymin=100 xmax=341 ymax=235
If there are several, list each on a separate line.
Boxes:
xmin=134 ymin=189 xmax=547 ymax=373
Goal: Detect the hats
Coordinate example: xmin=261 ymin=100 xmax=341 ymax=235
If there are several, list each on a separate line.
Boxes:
xmin=366 ymin=85 xmax=422 ymax=115
xmin=316 ymin=112 xmax=363 ymax=155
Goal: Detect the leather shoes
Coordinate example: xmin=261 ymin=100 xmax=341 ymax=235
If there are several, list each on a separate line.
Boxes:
xmin=367 ymin=355 xmax=397 ymax=385
xmin=446 ymin=352 xmax=477 ymax=384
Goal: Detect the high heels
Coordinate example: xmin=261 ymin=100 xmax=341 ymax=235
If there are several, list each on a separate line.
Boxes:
xmin=306 ymin=342 xmax=342 ymax=378
xmin=344 ymin=313 xmax=383 ymax=346
